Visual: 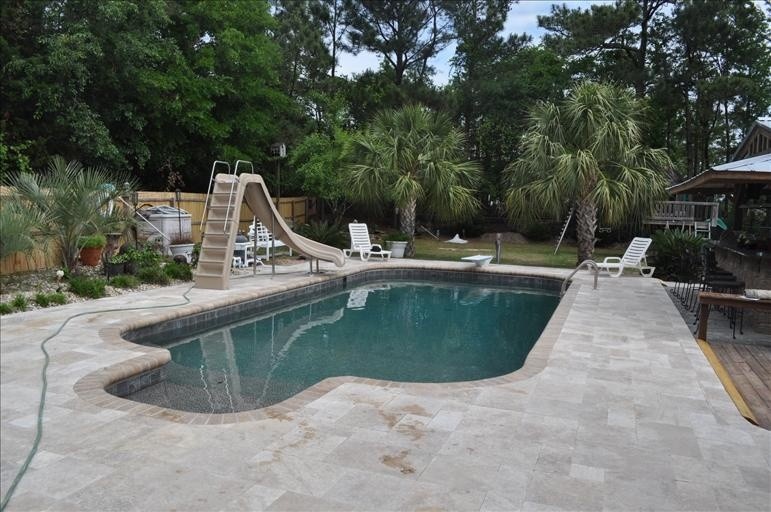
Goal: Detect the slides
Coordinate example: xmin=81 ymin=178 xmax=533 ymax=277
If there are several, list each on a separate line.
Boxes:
xmin=245 ymin=173 xmax=345 ymax=266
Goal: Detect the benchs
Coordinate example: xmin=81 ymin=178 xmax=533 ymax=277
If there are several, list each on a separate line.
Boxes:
xmin=250 ymin=216 xmax=292 ymax=263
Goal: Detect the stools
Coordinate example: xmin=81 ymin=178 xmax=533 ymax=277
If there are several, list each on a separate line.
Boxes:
xmin=232 ymin=257 xmax=241 ymax=267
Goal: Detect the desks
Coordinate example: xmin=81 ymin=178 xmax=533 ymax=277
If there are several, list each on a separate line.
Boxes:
xmin=234 ymin=241 xmax=269 ymax=267
xmin=697 ymin=290 xmax=771 ymax=340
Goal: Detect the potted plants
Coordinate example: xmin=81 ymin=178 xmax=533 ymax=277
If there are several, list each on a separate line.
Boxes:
xmin=383 ymin=230 xmax=411 ymax=258
xmin=76 ymin=203 xmax=147 ymax=275
xmin=167 ymin=235 xmax=195 ymax=264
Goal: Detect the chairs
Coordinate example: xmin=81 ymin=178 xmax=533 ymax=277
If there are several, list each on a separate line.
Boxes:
xmin=342 ymin=222 xmax=392 ymax=261
xmin=669 ymin=238 xmax=745 ymax=340
xmin=347 ymin=284 xmax=391 ymax=311
xmin=587 ymin=236 xmax=657 ymax=278
